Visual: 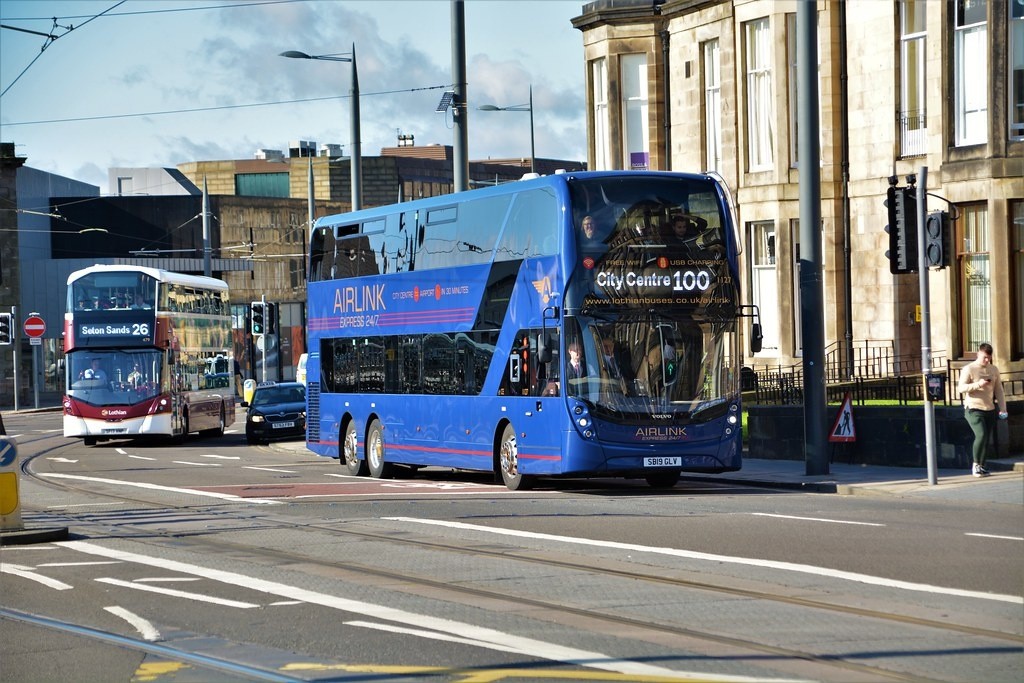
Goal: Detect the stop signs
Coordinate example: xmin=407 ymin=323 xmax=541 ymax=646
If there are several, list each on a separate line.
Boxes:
xmin=23 ymin=316 xmax=46 ymax=338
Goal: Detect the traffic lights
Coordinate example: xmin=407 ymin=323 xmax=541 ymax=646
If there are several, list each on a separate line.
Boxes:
xmin=250 ymin=301 xmax=265 ymax=336
xmin=923 ymin=212 xmax=953 ymax=268
xmin=0 ymin=312 xmax=12 ymax=346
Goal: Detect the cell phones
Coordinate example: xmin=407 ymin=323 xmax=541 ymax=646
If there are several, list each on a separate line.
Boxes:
xmin=986 ymin=379 xmax=991 ymax=382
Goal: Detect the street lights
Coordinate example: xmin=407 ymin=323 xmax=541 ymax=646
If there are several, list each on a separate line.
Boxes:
xmin=476 ymin=83 xmax=535 ymax=172
xmin=277 ymin=40 xmax=364 ymax=211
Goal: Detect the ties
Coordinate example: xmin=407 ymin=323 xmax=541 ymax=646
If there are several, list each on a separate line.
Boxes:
xmin=575 ymin=364 xmax=579 ymax=371
xmin=610 ymin=357 xmax=616 ymax=372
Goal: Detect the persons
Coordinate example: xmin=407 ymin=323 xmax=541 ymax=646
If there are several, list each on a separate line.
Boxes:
xmin=958 ymin=343 xmax=1008 ymax=478
xmin=580 ymin=216 xmax=605 ymax=244
xmin=601 ymin=340 xmax=625 ymax=393
xmin=130 ymin=293 xmax=151 ymax=308
xmin=568 ymin=343 xmax=590 ymax=395
xmin=85 ymin=358 xmax=108 ymax=384
xmin=542 ymin=382 xmax=558 ymax=398
xmin=667 ymin=214 xmax=708 ymax=247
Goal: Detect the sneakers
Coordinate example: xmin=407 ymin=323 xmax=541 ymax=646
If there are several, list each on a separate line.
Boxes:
xmin=972 ymin=467 xmax=990 ymax=477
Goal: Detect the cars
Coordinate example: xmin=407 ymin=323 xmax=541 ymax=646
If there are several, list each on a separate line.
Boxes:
xmin=240 ymin=381 xmax=306 ymax=446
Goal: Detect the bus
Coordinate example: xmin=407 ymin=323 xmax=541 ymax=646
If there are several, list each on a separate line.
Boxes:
xmin=295 ymin=167 xmax=765 ymax=492
xmin=56 ymin=262 xmax=237 ymax=446
